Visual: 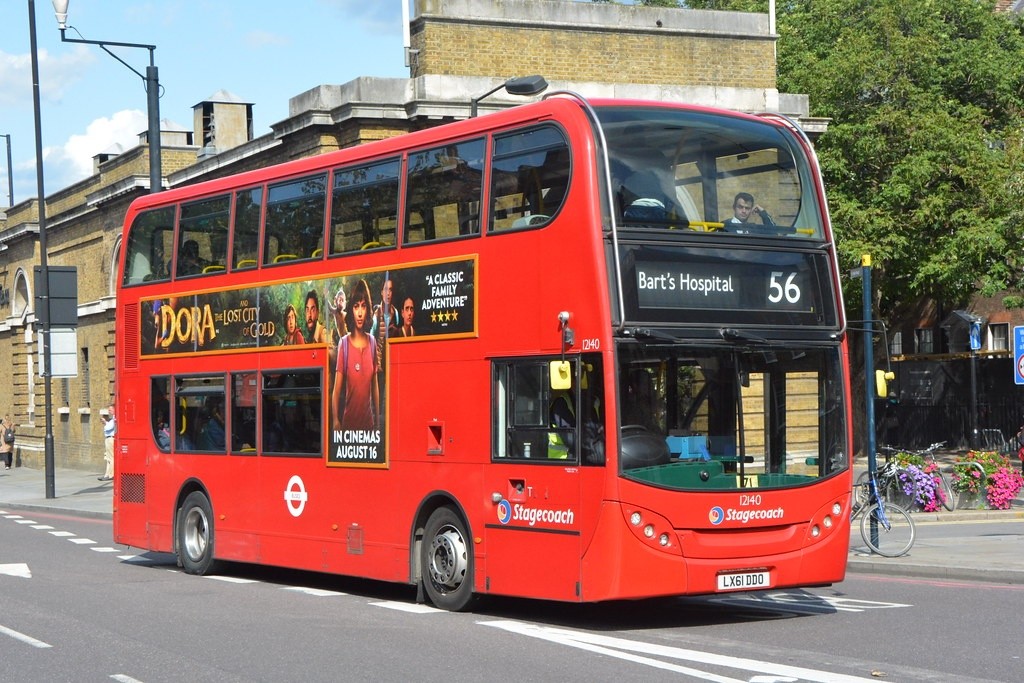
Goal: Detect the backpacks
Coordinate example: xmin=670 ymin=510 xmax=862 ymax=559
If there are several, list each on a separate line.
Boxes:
xmin=3 ymin=423 xmax=16 ymax=443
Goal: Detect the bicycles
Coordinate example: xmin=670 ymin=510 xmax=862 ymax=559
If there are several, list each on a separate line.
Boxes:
xmin=848 ymin=460 xmax=918 ymax=558
xmin=855 ymin=439 xmax=955 ymax=515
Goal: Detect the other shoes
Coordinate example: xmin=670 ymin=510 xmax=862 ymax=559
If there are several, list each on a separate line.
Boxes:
xmin=98 ymin=476 xmax=108 ymax=481
xmin=5 ymin=466 xmax=10 ymax=470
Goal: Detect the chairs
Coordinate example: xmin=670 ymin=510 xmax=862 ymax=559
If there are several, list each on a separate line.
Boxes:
xmin=144 ymin=240 xmax=392 ymax=281
xmin=512 ymin=214 xmax=550 ymax=230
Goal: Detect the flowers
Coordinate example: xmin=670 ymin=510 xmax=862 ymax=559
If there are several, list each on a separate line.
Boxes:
xmin=887 ymin=448 xmax=948 ymax=515
xmin=950 ymin=449 xmax=1024 ymax=511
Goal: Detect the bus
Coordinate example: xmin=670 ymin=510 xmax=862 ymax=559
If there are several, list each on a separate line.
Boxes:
xmin=112 ymin=88 xmax=895 ymax=612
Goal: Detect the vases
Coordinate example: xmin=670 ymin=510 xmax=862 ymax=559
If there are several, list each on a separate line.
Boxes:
xmin=889 ymin=485 xmax=923 ymax=514
xmin=958 ymin=483 xmax=994 ymax=511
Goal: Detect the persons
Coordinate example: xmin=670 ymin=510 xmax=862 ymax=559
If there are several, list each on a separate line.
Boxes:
xmin=548 ymin=361 xmax=605 ymax=465
xmin=158 ymin=397 xmax=252 ymax=451
xmin=168 ymin=240 xmax=214 ymax=276
xmin=610 ymin=128 xmax=673 ymax=227
xmin=715 ymin=190 xmax=779 ymax=235
xmin=0 ymin=414 xmax=16 ymax=471
xmin=282 ymin=279 xmax=415 ymax=465
xmin=99 ymin=406 xmax=114 ymax=481
